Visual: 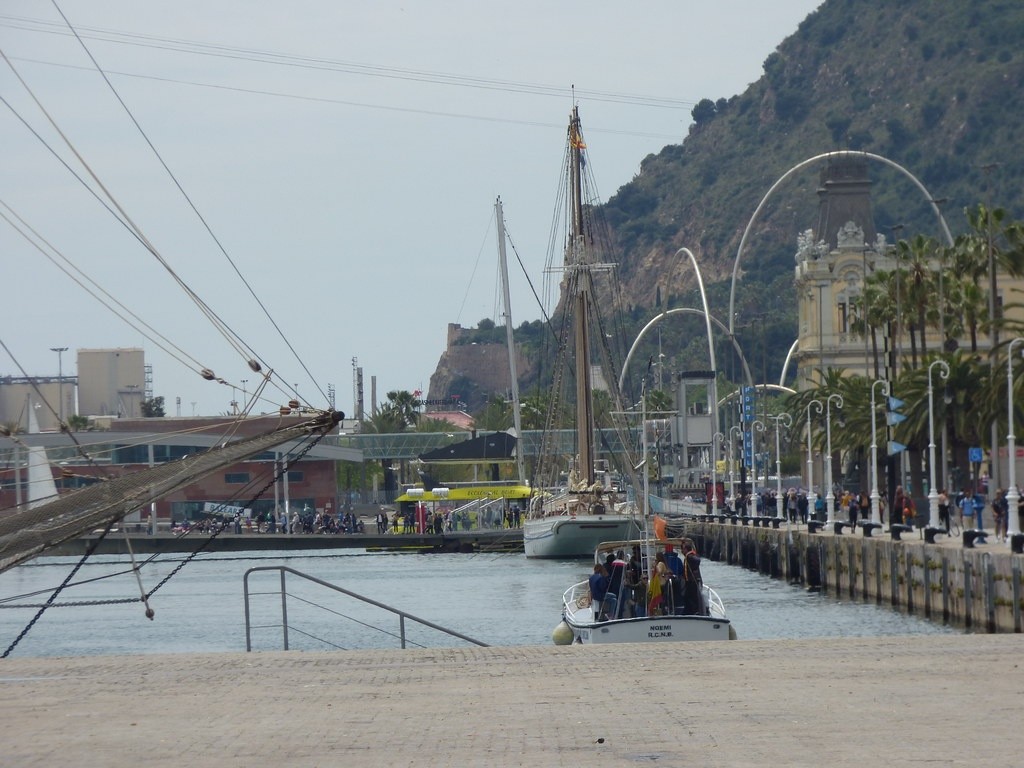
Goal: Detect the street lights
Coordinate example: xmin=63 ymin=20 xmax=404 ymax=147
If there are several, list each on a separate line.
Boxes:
xmin=869 ymin=379 xmax=890 ymax=538
xmin=751 ymin=419 xmax=767 ymax=528
xmin=711 ymin=432 xmax=725 ymax=522
xmin=1006 ymin=337 xmax=1024 ymax=552
xmin=775 ymin=412 xmax=793 ymax=521
xmin=808 ymin=400 xmax=824 ymax=521
xmin=823 ymin=393 xmax=844 ymax=532
xmin=927 ymin=360 xmax=952 ymax=542
xmin=728 ymin=424 xmax=744 ymax=518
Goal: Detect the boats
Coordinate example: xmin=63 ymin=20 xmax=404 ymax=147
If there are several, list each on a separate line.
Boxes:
xmin=551 ymin=378 xmax=736 ymax=645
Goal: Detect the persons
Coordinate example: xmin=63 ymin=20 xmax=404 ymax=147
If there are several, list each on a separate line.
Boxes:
xmin=611 ymin=551 xmax=628 ymax=570
xmin=685 ymin=492 xmax=692 ymax=502
xmin=590 ymin=564 xmax=617 ymax=621
xmin=625 ymin=573 xmax=651 ymax=617
xmin=630 ymin=546 xmax=647 ymax=573
xmin=147 ymin=504 xmax=520 ymax=535
xmin=682 ymin=541 xmax=700 ymax=615
xmin=725 ymin=485 xmax=1024 ymax=543
xmin=605 ymin=554 xmax=615 ymax=573
xmin=656 ymin=552 xmax=668 ymax=570
xmin=664 ymin=543 xmax=683 ymax=577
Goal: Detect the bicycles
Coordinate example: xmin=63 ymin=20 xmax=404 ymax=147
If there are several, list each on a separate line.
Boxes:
xmin=936 ymin=508 xmax=961 ymax=539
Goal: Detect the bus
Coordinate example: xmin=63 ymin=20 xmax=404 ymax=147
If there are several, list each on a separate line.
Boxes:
xmin=389 ymin=486 xmax=554 ymax=535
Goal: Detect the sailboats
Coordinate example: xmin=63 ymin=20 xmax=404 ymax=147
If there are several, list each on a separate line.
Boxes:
xmin=526 ymin=84 xmax=666 ymax=559
